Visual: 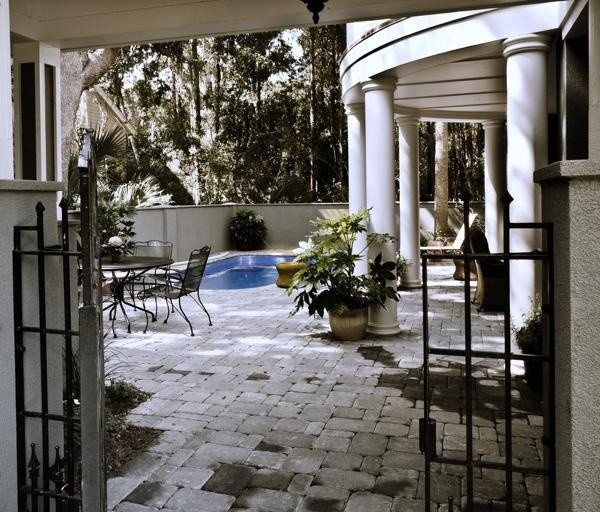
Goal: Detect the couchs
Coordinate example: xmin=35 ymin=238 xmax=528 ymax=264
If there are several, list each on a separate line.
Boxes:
xmin=467 ymin=228 xmax=509 ymax=312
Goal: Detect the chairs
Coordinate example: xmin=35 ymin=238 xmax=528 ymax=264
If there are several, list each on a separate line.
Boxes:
xmin=420 ymin=213 xmax=481 ymax=257
xmin=72 ymin=240 xmax=215 ymax=337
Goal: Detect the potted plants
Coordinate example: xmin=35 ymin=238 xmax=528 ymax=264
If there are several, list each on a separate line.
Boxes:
xmin=425 ymin=213 xmax=454 ymax=262
xmin=277 ymin=206 xmax=401 ymax=344
xmin=512 ymin=292 xmax=549 ymax=379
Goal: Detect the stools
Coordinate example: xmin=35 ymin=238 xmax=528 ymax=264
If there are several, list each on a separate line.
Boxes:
xmin=276 ymin=260 xmax=308 ymax=288
